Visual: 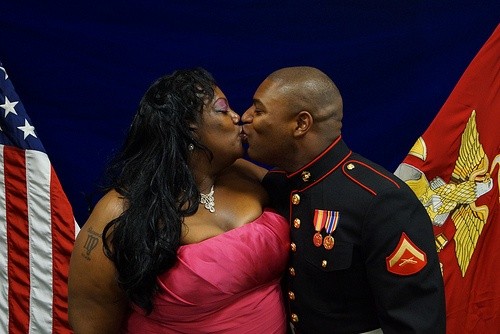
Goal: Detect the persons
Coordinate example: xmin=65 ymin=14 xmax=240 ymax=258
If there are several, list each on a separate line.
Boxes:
xmin=239 ymin=66 xmax=447 ymax=334
xmin=67 ymin=68 xmax=293 ymax=334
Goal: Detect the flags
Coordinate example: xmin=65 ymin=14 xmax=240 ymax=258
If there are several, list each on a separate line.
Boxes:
xmin=391 ymin=26 xmax=499 ymax=332
xmin=0 ymin=64 xmax=88 ymax=334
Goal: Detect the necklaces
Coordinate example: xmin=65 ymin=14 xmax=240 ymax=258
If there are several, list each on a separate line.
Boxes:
xmin=181 ymin=182 xmax=216 ymax=214
